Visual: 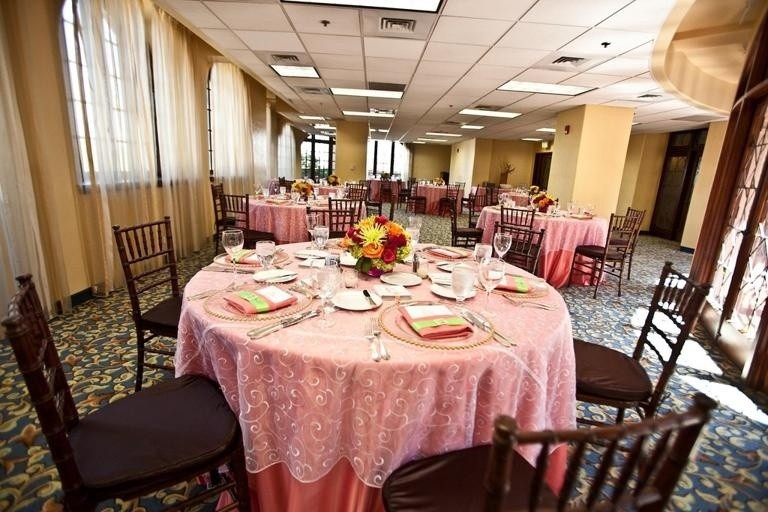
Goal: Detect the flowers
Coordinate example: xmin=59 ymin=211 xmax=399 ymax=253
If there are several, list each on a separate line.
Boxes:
xmin=342 ymin=216 xmax=411 ymax=273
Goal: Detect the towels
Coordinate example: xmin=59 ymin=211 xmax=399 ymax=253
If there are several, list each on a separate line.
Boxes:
xmin=495 ymin=276 xmax=533 ymax=292
xmin=223 ymin=284 xmax=297 ymax=316
xmin=398 ymin=304 xmax=474 ymax=340
xmin=429 ymin=247 xmax=471 ymax=259
xmin=225 ymin=251 xmax=266 ymax=264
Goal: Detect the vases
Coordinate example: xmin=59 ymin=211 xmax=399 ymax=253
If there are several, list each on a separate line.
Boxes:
xmin=368 ymin=267 xmax=393 ymax=277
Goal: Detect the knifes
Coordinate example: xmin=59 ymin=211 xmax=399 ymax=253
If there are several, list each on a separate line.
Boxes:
xmin=462 ymin=309 xmax=521 ymax=352
xmin=244 ymin=310 xmax=320 ymax=341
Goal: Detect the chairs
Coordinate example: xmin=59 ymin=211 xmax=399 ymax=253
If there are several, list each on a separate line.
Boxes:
xmin=576 ymin=260 xmax=714 ymax=459
xmin=0 ymin=272 xmax=252 ymax=511
xmin=383 ymin=391 xmax=719 ymax=511
xmin=112 ymin=213 xmax=186 ymax=390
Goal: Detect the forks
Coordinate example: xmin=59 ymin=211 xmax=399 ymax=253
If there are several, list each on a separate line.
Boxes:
xmin=364 ymin=314 xmax=390 ymax=363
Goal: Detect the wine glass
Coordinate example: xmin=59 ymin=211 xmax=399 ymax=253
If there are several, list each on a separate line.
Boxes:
xmin=205 ymin=212 xmax=557 ymax=307
xmin=498 ymin=190 xmax=598 ymax=220
xmin=259 ymin=181 xmax=344 ymax=205
